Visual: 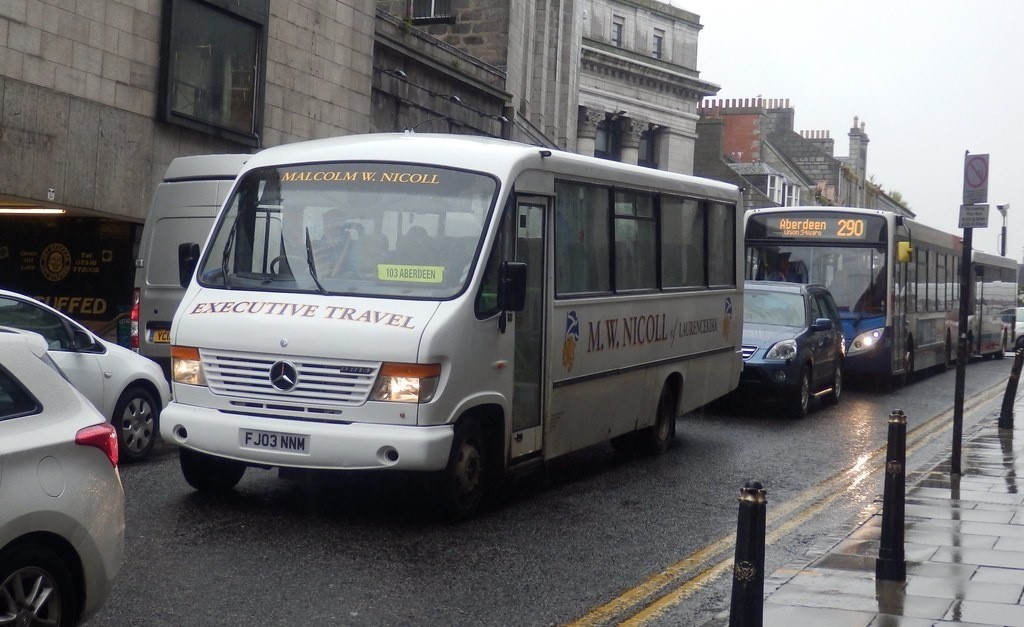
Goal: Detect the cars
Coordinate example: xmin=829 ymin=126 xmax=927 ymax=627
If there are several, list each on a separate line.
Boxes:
xmin=1005 ymin=307 xmax=1024 ymax=352
xmin=0 ymin=326 xmax=126 ymax=627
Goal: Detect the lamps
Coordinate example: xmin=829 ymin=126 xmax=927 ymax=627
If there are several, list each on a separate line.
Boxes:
xmin=480 ymin=112 xmax=510 ymax=123
xmin=428 ymin=92 xmax=462 ymax=103
xmin=375 ymin=67 xmax=408 ymax=78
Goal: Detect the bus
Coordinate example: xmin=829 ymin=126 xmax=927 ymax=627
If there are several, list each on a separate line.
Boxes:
xmin=159 ymin=130 xmax=745 ymax=525
xmin=0 ymin=289 xmax=170 ymax=461
xmin=743 ymin=206 xmax=1020 ymax=387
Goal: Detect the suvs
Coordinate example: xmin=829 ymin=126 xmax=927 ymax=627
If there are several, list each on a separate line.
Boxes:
xmin=733 ymin=280 xmax=846 ymax=418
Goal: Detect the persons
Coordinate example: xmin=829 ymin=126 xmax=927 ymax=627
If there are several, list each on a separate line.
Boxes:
xmin=766 ymin=254 xmax=800 ymax=282
xmin=303 ymin=209 xmax=354 ymax=276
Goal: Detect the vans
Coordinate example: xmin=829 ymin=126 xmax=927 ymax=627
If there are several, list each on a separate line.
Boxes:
xmin=131 ymin=154 xmax=486 ymax=401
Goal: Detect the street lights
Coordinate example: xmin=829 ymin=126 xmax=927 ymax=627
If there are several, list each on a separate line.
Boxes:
xmin=997 ymin=203 xmax=1009 ymax=257
xmin=410 ymin=117 xmax=465 ymax=133
